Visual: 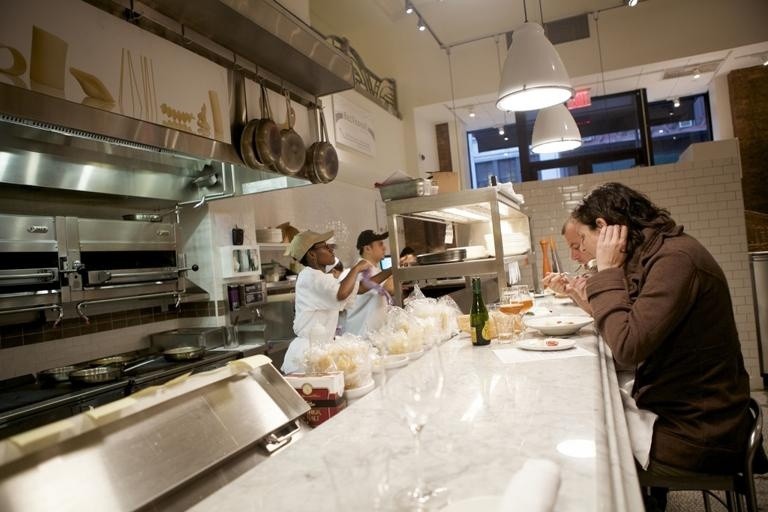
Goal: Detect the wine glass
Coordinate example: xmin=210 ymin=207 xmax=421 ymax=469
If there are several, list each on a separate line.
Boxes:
xmin=499 ymin=287 xmax=525 ymax=334
xmin=382 ymin=342 xmax=451 ymax=512
xmin=515 ymin=284 xmax=534 ymax=316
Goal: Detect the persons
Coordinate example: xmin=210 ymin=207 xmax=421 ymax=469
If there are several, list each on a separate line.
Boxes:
xmin=571 ymin=182 xmax=751 ymax=488
xmin=541 ymin=215 xmax=595 ymax=319
xmin=280 ymin=230 xmax=417 ymax=375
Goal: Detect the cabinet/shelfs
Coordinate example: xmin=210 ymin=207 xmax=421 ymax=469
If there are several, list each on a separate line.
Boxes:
xmin=385 ymin=188 xmax=537 ymax=308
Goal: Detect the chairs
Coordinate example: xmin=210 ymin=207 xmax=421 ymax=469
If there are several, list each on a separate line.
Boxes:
xmin=642 ymin=396 xmax=763 ymax=511
xmin=699 ymin=484 xmax=733 ymax=511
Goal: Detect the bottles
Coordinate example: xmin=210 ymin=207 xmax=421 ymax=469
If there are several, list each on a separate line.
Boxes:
xmin=470 ymin=276 xmax=492 ymax=346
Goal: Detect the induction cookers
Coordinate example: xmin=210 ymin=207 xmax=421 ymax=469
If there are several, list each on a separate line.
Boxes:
xmin=76 ymin=347 xmax=238 ymax=384
xmin=1 ymin=374 xmax=129 ymax=411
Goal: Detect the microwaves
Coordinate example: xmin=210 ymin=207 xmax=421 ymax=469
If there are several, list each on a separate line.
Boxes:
xmin=238 ymin=281 xmax=267 ymax=305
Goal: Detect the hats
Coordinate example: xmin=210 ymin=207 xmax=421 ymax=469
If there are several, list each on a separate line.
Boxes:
xmin=356 ymin=230 xmax=390 ymax=250
xmin=282 ymin=230 xmax=335 ymax=262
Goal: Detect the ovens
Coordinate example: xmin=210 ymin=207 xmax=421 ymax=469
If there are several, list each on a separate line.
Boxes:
xmin=0 ymin=216 xmax=65 ymax=317
xmin=68 ymin=216 xmax=198 ymax=302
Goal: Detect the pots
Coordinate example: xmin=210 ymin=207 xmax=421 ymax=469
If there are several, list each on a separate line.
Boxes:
xmin=238 ymin=81 xmax=339 ymax=185
xmin=40 ymin=356 xmax=156 ymax=384
xmin=164 ymin=344 xmax=206 ymax=360
xmin=123 ymin=214 xmax=162 ymax=221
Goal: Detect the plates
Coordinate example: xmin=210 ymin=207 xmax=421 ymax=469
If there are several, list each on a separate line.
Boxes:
xmin=417 ymin=249 xmax=467 ymax=265
xmin=384 ymin=346 xmax=427 ymax=369
xmin=515 ymin=336 xmax=577 ymax=351
xmin=525 ymin=316 xmax=593 ymax=337
xmin=256 ymin=228 xmax=282 ymax=243
xmin=346 ymin=379 xmax=377 ymax=398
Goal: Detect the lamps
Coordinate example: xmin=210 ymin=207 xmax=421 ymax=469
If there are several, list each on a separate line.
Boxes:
xmin=625 ymin=0 xmax=638 ymax=8
xmin=404 ymin=1 xmax=414 ymax=14
xmin=529 ymin=102 xmax=584 ymax=154
xmin=493 ymin=0 xmax=575 ymax=112
xmin=417 ymin=18 xmax=426 ymax=32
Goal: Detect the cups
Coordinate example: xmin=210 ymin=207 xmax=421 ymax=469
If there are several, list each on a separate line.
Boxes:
xmin=494 ymin=312 xmax=515 ymax=344
